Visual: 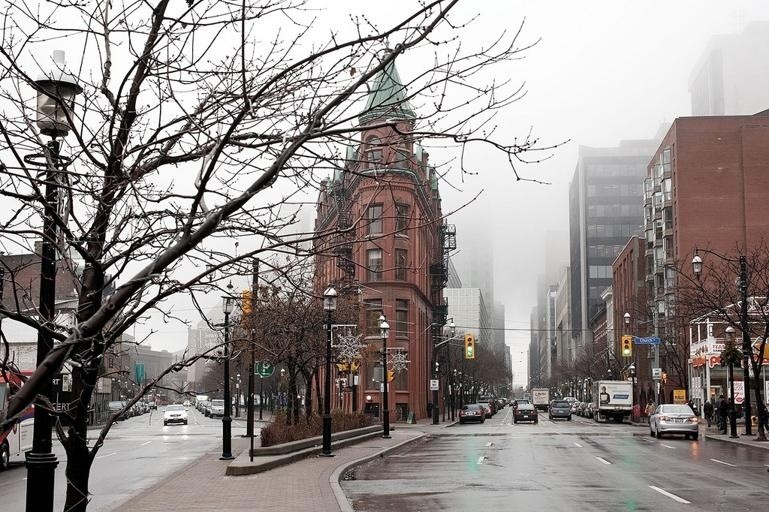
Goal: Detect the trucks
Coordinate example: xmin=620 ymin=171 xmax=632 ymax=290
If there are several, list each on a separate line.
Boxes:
xmin=593 ymin=379 xmax=633 ymax=423
xmin=0 ymin=369 xmax=37 ymax=471
xmin=532 ymin=387 xmax=549 ymax=412
xmin=528 ymin=395 xmax=533 ymax=404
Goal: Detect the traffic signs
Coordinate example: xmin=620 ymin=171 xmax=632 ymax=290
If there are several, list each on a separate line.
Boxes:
xmin=634 ymin=337 xmax=661 ymax=345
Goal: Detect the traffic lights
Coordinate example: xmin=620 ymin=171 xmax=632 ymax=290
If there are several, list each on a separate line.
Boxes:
xmin=388 ymin=370 xmax=394 ymax=382
xmin=242 ymin=290 xmax=254 ymax=317
xmin=753 ymin=342 xmax=769 ymax=359
xmin=337 ymin=364 xmax=345 ymax=371
xmin=629 ymin=365 xmax=636 ymax=422
xmin=109 ymin=358 xmax=113 ymax=367
xmin=465 ymin=334 xmax=475 ymax=359
xmin=351 ymin=364 xmax=358 ymax=372
xmin=622 ymin=336 xmax=632 ymax=357
xmin=663 ymin=373 xmax=668 ymax=384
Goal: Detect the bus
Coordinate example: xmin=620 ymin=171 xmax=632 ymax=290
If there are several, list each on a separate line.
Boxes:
xmin=524 ymin=392 xmax=530 ymax=399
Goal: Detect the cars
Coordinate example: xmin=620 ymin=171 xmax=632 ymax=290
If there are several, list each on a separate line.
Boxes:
xmin=460 ymin=397 xmax=538 ymax=424
xmin=648 ymin=403 xmax=698 ymax=441
xmin=108 ymin=398 xmax=158 ymax=420
xmin=162 ymin=405 xmax=190 ymax=425
xmin=183 ymin=395 xmax=226 ymax=418
xmin=563 ymin=395 xmax=593 ymax=419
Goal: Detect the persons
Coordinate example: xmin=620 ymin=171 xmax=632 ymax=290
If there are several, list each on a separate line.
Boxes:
xmin=741 ymin=397 xmax=747 ymax=427
xmin=427 ymin=401 xmax=433 ymax=418
xmin=715 ymin=395 xmax=732 ymax=435
xmin=762 ymin=400 xmax=769 ymax=434
xmin=600 ymin=386 xmax=610 ymax=404
xmin=645 ymin=399 xmax=655 ymax=427
xmin=633 ymin=402 xmax=641 ymax=422
xmin=688 ymin=400 xmax=694 ymax=414
xmin=704 ymin=400 xmax=714 ymax=427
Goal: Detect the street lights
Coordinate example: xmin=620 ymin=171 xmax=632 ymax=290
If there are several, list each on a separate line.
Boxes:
xmin=624 ymin=312 xmax=659 ymax=407
xmin=206 ymin=249 xmax=260 ymax=439
xmin=111 ymin=377 xmax=156 ymax=400
xmin=220 ymin=278 xmax=237 ymax=461
xmin=724 ymin=326 xmax=740 ymax=439
xmin=231 ymin=373 xmax=241 ymax=418
xmin=551 ymin=369 xmax=612 ymax=401
xmin=434 ymin=360 xmax=440 ymax=424
xmin=260 ymin=374 xmax=264 ymax=420
xmin=322 ymin=285 xmax=339 ymax=458
xmin=692 ymin=246 xmax=755 ymax=436
xmin=451 ymin=367 xmax=498 ymax=421
xmin=280 ymin=369 xmax=286 ymax=424
xmin=379 ymin=314 xmax=392 ymax=439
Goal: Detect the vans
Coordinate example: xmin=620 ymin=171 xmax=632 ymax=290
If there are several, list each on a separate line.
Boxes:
xmin=547 ymin=400 xmax=571 ymax=421
